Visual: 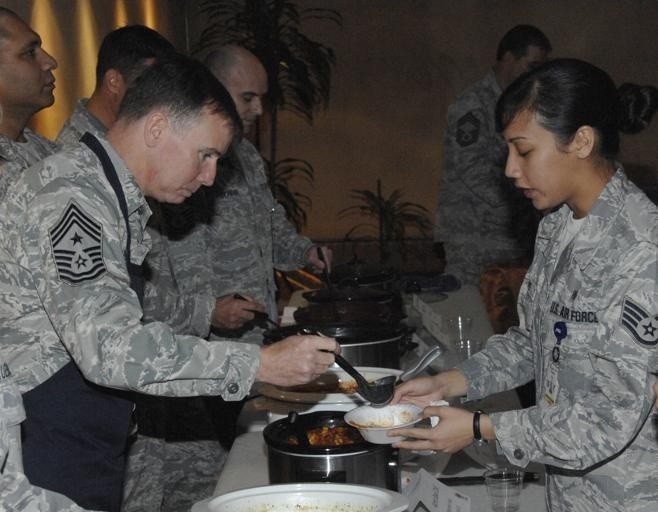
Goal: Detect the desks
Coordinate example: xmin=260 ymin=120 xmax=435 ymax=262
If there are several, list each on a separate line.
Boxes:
xmin=212 ymin=267 xmax=555 ymax=512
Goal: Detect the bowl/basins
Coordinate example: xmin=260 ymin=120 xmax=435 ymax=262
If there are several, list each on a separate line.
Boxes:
xmin=342 ymin=404 xmax=424 ymax=445
xmin=192 ymin=481 xmax=412 ymax=512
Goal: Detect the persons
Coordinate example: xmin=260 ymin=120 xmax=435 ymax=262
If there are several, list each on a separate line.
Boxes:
xmin=0 ymin=57 xmax=342 ymax=512
xmin=434 ymin=24 xmax=556 ymax=284
xmin=53 ymin=23 xmax=266 ymax=338
xmin=123 ymin=34 xmax=333 ymax=511
xmin=0 ymin=5 xmax=64 ymax=189
xmin=391 ymin=61 xmax=658 ymax=512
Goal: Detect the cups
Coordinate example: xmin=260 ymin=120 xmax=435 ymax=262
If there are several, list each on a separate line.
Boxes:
xmin=443 ymin=313 xmax=472 ymax=349
xmin=451 ymin=337 xmax=483 ymax=362
xmin=481 ymin=467 xmax=522 ymax=512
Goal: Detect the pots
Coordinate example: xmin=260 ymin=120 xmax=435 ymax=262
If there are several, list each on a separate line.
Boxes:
xmin=263 ymin=255 xmax=407 ymax=401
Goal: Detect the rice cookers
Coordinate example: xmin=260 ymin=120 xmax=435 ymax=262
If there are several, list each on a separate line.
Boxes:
xmin=260 ymin=410 xmax=405 ymax=496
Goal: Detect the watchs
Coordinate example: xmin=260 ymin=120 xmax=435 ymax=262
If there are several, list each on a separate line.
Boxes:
xmin=472 ymin=410 xmax=489 ymax=447
